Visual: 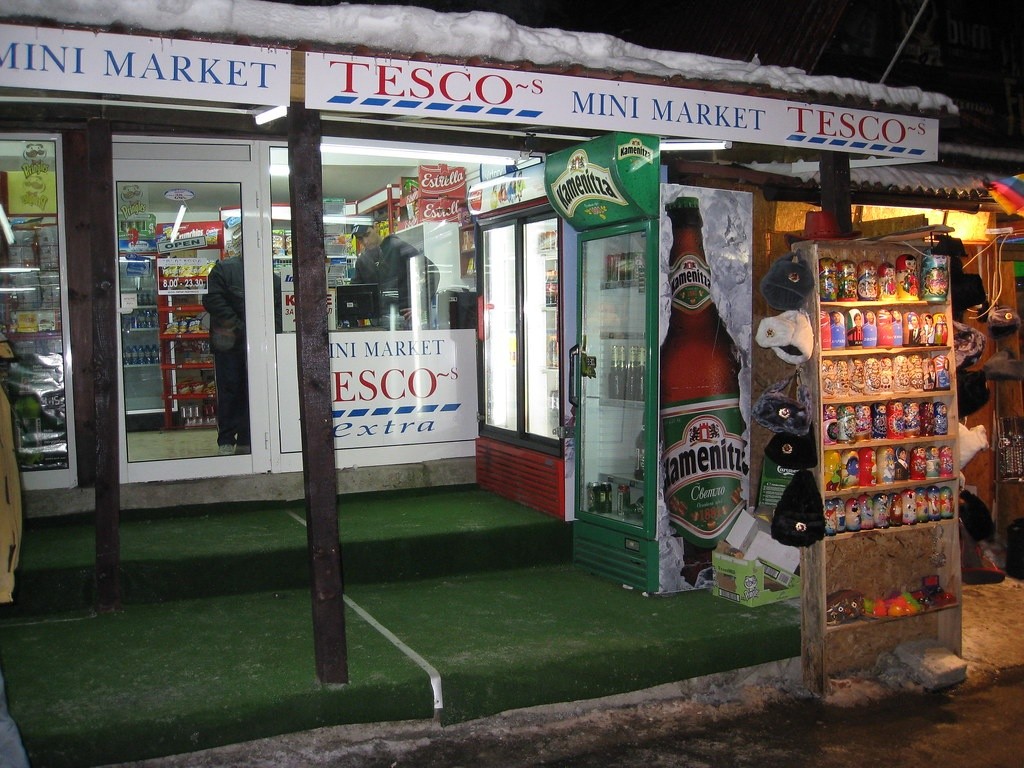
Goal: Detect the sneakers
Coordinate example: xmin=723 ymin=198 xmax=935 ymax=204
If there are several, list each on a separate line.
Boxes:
xmin=218 ymin=444 xmax=234 ymax=456
xmin=235 ymin=445 xmax=250 ymax=454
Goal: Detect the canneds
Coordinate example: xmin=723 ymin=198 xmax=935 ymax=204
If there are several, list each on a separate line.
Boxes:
xmin=137 ymin=293 xmax=154 ymax=305
xmin=606 ymin=251 xmax=643 ymax=281
xmin=618 ymin=485 xmax=630 ymax=515
xmin=589 ymin=481 xmax=600 ymax=512
xmin=538 ymin=229 xmax=557 ymax=250
xmin=181 ymin=405 xmax=216 ymax=424
xmin=600 ymin=482 xmax=612 ymax=513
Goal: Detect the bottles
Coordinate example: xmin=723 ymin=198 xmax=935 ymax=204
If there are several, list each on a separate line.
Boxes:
xmin=659 ymin=196 xmax=749 ymax=590
xmin=121 ymin=308 xmax=158 ymax=329
xmin=549 ymin=384 xmax=559 ymax=428
xmin=602 ymin=253 xmax=644 ymax=293
xmin=123 ymin=344 xmax=159 ymax=365
xmin=510 ymin=331 xmax=516 ymax=366
xmin=609 ymin=345 xmax=644 ymax=400
xmin=547 ymin=336 xmax=559 ymax=370
xmin=633 ymin=411 xmax=645 ymax=481
xmin=545 ymin=263 xmax=558 ymax=308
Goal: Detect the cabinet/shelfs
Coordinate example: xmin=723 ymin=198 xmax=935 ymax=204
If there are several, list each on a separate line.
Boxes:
xmin=220 ymin=208 xmax=372 ymax=289
xmin=395 ymin=221 xmax=461 ymax=329
xmin=0 ymin=224 xmax=62 ymax=341
xmin=792 ymin=238 xmax=963 ymax=697
xmin=159 ymin=276 xmax=220 ymax=429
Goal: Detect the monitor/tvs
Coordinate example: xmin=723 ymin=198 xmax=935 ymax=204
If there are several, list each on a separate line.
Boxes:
xmin=337 ymin=284 xmax=380 ymax=322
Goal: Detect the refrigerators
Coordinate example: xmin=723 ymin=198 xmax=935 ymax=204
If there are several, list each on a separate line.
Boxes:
xmin=467 ymin=133 xmax=752 ymax=596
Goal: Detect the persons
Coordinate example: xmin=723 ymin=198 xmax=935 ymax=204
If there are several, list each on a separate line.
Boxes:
xmin=351 ymin=219 xmax=440 ymax=330
xmin=202 ymin=232 xmax=252 ymax=457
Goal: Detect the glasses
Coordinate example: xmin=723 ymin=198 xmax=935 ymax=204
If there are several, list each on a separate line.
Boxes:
xmin=356 ymin=231 xmax=370 ymax=240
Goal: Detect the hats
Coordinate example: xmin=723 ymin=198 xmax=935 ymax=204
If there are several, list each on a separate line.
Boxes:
xmin=952 ymin=319 xmax=986 ymax=370
xmin=350 ymin=213 xmax=373 ymax=238
xmin=772 ymin=470 xmax=825 ymax=547
xmin=750 ymin=370 xmax=812 ymax=436
xmin=959 ymin=489 xmax=995 ymax=542
xmin=758 ymin=253 xmax=813 ymax=310
xmin=953 ymin=273 xmax=986 ymax=310
xmin=957 ymin=368 xmax=990 ymax=419
xmin=982 ymin=348 xmax=1023 ymax=381
xmin=957 ymin=422 xmax=990 ymax=470
xmin=769 ymin=426 xmax=818 ymax=470
xmin=987 ymin=305 xmax=1020 ymax=340
xmin=755 ymin=310 xmax=814 ymax=363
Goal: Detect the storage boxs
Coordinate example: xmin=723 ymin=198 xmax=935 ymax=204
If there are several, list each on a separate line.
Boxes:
xmin=400 ymin=165 xmax=467 ymax=224
